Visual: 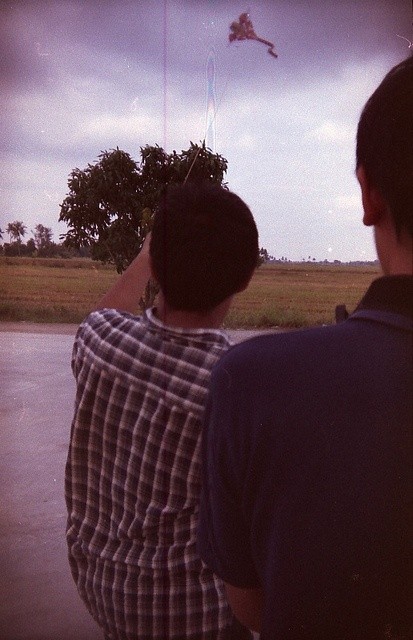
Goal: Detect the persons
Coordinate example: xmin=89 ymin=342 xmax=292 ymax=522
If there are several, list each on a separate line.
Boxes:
xmin=64 ymin=180 xmax=264 ymax=640
xmin=196 ymin=55 xmax=413 ymax=640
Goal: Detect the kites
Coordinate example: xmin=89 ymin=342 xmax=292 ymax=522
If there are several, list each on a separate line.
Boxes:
xmin=229 ymin=13 xmax=277 ymax=58
